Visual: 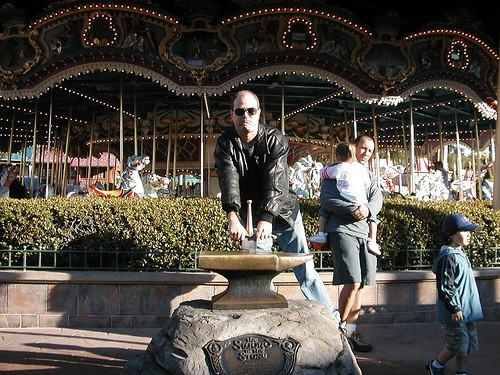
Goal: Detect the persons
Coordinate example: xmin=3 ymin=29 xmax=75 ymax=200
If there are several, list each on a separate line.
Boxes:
xmin=320 ymin=134 xmax=383 ymax=352
xmin=1 ymin=166 xmax=28 ymax=199
xmin=213 ymin=90 xmax=354 ymax=351
xmin=307 ymin=142 xmax=381 ymax=255
xmin=424 ymin=213 xmax=485 ymax=375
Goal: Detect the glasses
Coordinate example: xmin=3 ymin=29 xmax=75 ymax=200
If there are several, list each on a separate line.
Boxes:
xmin=232 ymin=107 xmax=260 ymax=116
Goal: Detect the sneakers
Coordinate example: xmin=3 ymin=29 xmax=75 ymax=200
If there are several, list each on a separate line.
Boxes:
xmin=425 ymin=360 xmax=447 ymax=374
xmin=348 ymin=330 xmax=375 ymax=352
xmin=367 ymin=242 xmax=382 ymax=255
xmin=306 ymin=234 xmax=327 ymax=244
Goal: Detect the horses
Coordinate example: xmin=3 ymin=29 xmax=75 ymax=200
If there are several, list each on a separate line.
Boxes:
xmin=373 ymin=165 xmax=405 ymax=197
xmin=67 ymin=154 xmax=169 ymax=199
xmin=288 ymin=155 xmax=323 ymax=200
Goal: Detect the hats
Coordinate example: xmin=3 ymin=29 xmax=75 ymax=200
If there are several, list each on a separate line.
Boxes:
xmin=442 ymin=213 xmax=479 ymax=233
